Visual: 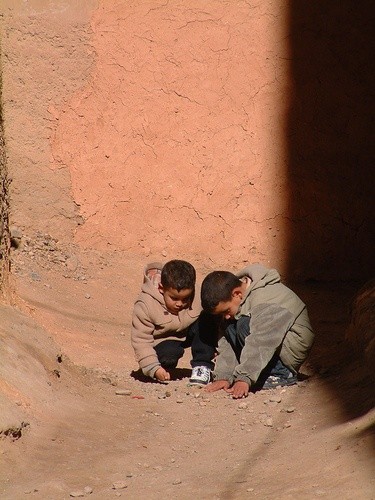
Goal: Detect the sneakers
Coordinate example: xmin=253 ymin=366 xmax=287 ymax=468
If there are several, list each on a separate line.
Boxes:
xmin=189 ymin=365 xmax=214 ymax=385
xmin=261 ymin=370 xmax=297 ymax=390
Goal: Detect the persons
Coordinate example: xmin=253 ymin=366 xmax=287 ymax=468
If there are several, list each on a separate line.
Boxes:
xmin=199 ymin=260 xmax=309 ymax=399
xmin=129 ymin=256 xmax=224 ymax=384
